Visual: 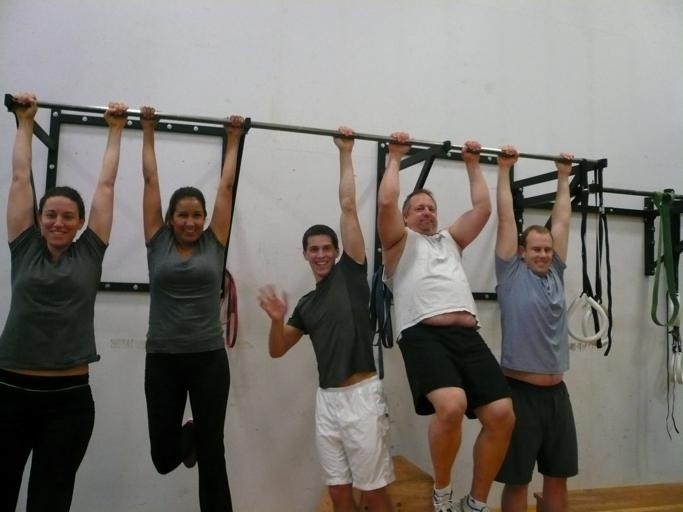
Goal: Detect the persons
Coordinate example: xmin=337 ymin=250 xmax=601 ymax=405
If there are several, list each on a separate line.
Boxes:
xmin=139 ymin=106 xmax=245 ymax=512
xmin=258 ymin=127 xmax=397 ymax=512
xmin=496 ymin=144 xmax=579 ymax=511
xmin=0 ymin=92 xmax=128 ymax=512
xmin=378 ymin=132 xmax=518 ymax=512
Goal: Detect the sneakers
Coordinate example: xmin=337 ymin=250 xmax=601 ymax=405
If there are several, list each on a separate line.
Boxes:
xmin=462 ymin=494 xmax=490 ymax=511
xmin=431 ymin=489 xmax=458 ymax=512
